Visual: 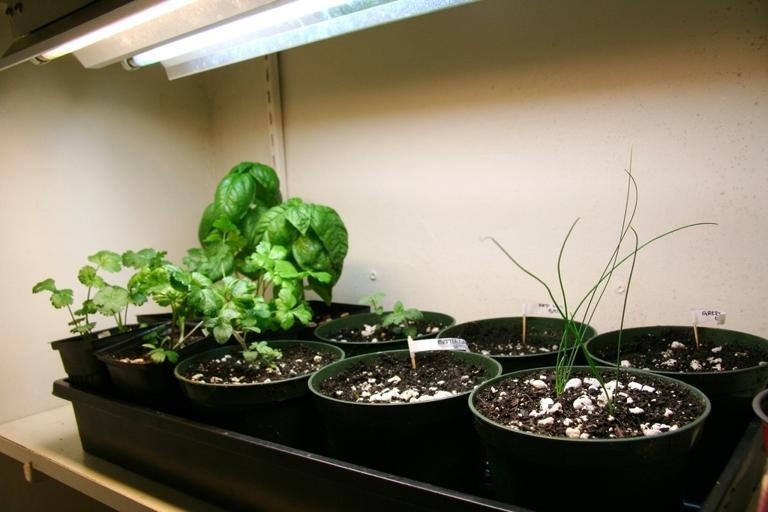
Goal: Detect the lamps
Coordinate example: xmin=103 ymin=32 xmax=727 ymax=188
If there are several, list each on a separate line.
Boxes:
xmin=1 ymin=0 xmax=496 ymax=83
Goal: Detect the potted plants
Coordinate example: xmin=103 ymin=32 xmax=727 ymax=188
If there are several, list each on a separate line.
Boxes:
xmin=29 ymin=146 xmax=766 ymax=512
